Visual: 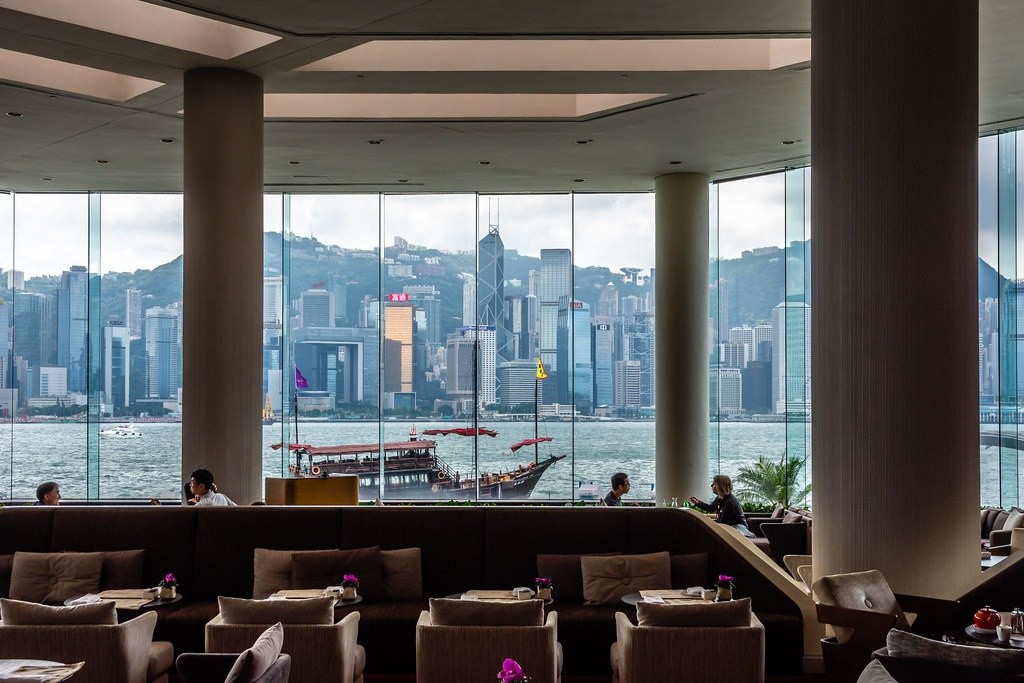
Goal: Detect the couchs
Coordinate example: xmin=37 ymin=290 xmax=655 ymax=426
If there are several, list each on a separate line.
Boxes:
xmin=0 ymin=609 xmax=1024 ymax=683
xmin=742 ymin=507 xmax=812 ymax=568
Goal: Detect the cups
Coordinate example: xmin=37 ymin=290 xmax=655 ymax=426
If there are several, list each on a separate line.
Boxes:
xmin=327 ymin=590 xmax=340 ymax=599
xmin=996 ymin=625 xmax=1012 ymax=641
xmin=518 ymin=590 xmax=531 ymax=600
xmin=143 ymin=588 xmax=158 ymax=599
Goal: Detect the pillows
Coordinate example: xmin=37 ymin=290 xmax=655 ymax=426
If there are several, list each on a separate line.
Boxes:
xmin=856 ymin=628 xmax=1024 ymax=683
xmin=770 ymin=506 xmax=784 ymax=518
xmin=781 ymin=512 xmax=802 ymax=524
xmin=0 ymin=546 xmax=751 ymax=683
xmin=980 ymin=509 xmax=1024 ymax=537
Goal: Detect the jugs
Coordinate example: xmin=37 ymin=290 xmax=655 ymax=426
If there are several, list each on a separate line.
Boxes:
xmin=1011 ymin=608 xmax=1024 ymax=635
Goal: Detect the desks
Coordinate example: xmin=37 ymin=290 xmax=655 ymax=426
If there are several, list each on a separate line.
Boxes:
xmin=965 ymin=624 xmax=1024 ymax=649
xmin=64 ymin=591 xmax=183 ymax=621
xmin=459 ymin=589 xmax=554 ymax=612
xmin=621 ymin=592 xmax=734 ymax=613
xmin=264 ymin=589 xmax=363 ymax=611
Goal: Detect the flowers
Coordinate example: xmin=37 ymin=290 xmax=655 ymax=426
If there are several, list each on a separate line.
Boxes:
xmin=534 ymin=578 xmax=552 ymax=591
xmin=158 ymin=572 xmax=179 ymax=590
xmin=717 ymin=574 xmax=736 ymax=590
xmin=341 ymin=575 xmax=359 ymax=589
xmin=497 ymin=658 xmax=525 ymax=683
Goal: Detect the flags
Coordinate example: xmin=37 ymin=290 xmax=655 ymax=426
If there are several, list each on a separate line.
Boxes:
xmin=295 ymin=368 xmax=308 ymax=389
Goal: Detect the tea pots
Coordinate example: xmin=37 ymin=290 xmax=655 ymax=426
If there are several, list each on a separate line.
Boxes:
xmin=974 ymin=605 xmax=1002 ymax=629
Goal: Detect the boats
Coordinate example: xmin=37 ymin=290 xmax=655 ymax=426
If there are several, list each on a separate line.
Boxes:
xmin=271 ymin=438 xmax=567 ymax=500
xmin=98 ymin=424 xmax=143 ymax=439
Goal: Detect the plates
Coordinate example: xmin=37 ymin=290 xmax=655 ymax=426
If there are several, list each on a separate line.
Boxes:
xmin=975 ymin=626 xmax=997 ymax=633
xmin=683 ymin=590 xmax=701 ymax=596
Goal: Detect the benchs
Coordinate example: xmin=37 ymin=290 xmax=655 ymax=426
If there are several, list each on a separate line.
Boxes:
xmin=981 ymin=508 xmax=1024 ymax=556
xmin=0 ymin=504 xmax=822 ymax=683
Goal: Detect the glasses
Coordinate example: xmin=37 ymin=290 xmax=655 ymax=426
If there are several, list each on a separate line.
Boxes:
xmin=622 ymin=483 xmax=630 ymax=488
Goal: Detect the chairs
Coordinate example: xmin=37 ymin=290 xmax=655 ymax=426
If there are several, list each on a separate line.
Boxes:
xmin=810 ymin=569 xmax=993 ymax=683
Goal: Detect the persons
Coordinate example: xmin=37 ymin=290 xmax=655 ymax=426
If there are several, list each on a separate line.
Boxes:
xmin=151 ymin=469 xmax=238 ymax=506
xmin=604 ymin=473 xmax=630 ymax=506
xmin=690 ymin=475 xmax=749 ymax=529
xmin=36 ymin=482 xmax=61 ymax=505
